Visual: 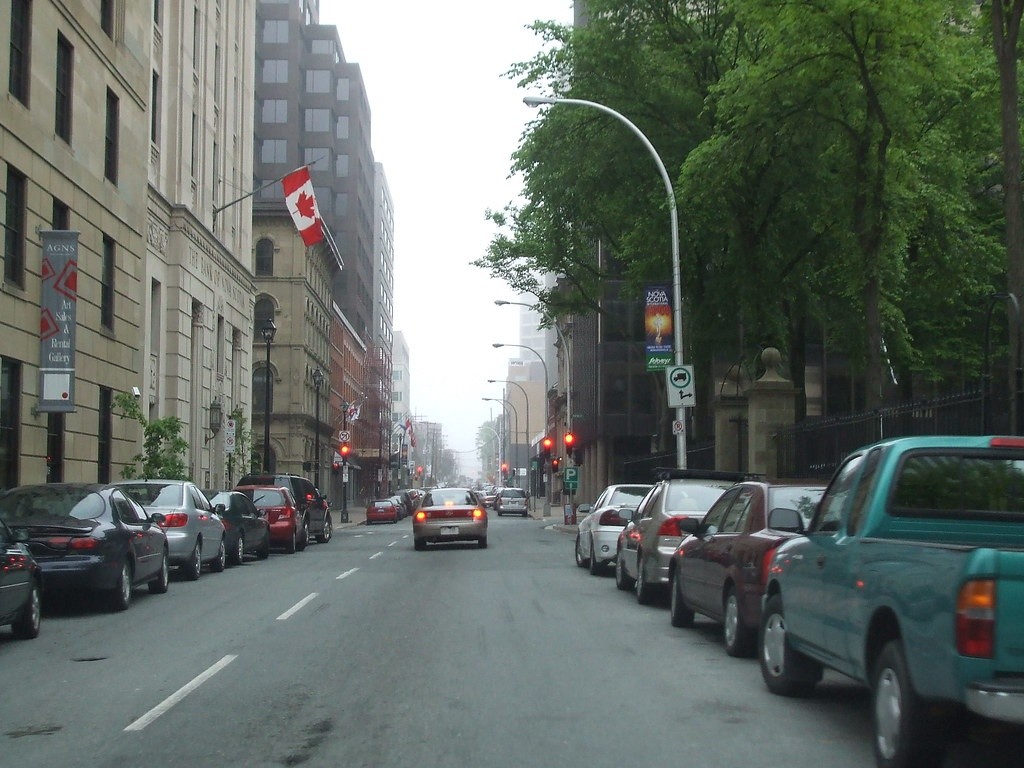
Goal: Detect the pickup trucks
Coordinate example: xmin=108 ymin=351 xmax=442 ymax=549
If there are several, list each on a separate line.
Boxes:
xmin=760 ymin=435 xmax=1024 ymax=768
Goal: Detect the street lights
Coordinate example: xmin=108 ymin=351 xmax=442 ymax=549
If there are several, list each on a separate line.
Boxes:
xmin=260 ymin=319 xmax=278 ymax=473
xmin=377 ymin=422 xmax=384 ymax=499
xmin=495 ymin=299 xmax=575 ymax=503
xmin=396 ymin=433 xmax=403 ymax=488
xmin=492 ymin=344 xmax=552 ymax=500
xmin=388 ymin=427 xmax=393 ymax=493
xmin=482 ymin=397 xmax=519 ymax=487
xmin=520 ymin=95 xmax=687 ymax=469
xmin=488 ymin=380 xmax=531 ymax=511
xmin=338 ymin=400 xmax=349 ymax=524
xmin=310 ymin=364 xmax=324 ymax=487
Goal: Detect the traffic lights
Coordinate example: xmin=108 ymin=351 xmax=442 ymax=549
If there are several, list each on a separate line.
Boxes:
xmin=341 ymin=444 xmax=348 ymax=455
xmin=544 ymin=438 xmax=551 ymax=458
xmin=552 ymin=460 xmax=559 ymax=472
xmin=564 ymin=434 xmax=573 ymax=444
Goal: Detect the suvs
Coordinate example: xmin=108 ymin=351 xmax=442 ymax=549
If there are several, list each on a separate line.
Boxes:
xmin=236 ymin=472 xmax=331 ymax=546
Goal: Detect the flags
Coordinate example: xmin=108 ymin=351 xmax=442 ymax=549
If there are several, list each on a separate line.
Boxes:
xmin=348 ymin=404 xmax=361 ymax=420
xmin=281 ymin=167 xmax=324 ymax=247
xmin=400 ymin=418 xmax=416 ymax=447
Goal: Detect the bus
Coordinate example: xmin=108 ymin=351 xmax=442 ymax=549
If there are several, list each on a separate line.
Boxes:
xmin=413 ymin=488 xmax=490 ymax=549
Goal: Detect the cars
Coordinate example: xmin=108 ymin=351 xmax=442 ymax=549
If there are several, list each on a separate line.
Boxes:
xmin=365 ymin=488 xmax=426 ymax=525
xmin=666 ymin=478 xmax=833 ymax=657
xmin=0 ymin=480 xmax=169 ymax=611
xmin=196 ymin=488 xmax=270 ymax=565
xmin=615 ymin=471 xmax=767 ymax=605
xmin=231 ymin=485 xmax=309 ymax=553
xmin=477 ymin=483 xmax=529 ymax=518
xmin=0 ymin=511 xmax=45 ymax=639
xmin=575 ymin=484 xmax=654 ymax=577
xmin=106 ymin=479 xmax=227 ymax=580
